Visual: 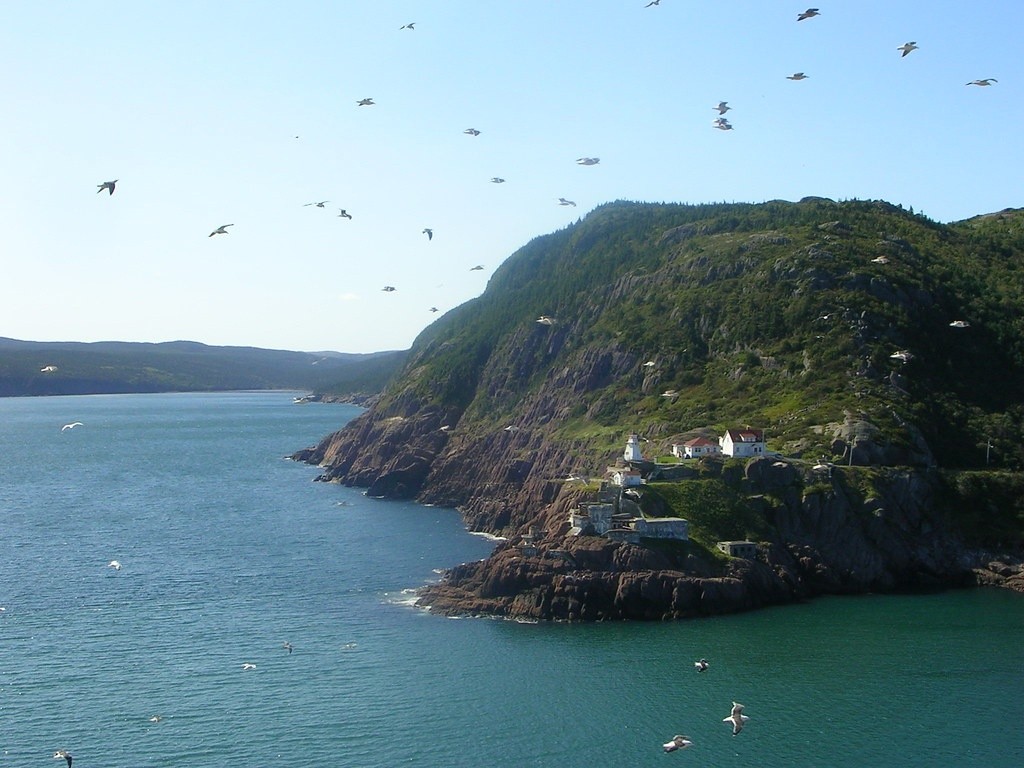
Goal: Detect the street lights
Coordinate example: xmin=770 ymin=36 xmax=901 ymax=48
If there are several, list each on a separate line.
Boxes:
xmin=848 ymin=435 xmax=853 ymax=467
xmin=987 ymin=438 xmax=991 ymax=464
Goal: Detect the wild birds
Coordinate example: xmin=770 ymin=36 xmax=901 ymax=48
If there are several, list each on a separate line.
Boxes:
xmin=503 ymin=426 xmax=520 ymax=435
xmin=149 ymin=713 xmax=162 ymax=724
xmin=208 ymin=223 xmax=234 ymax=238
xmin=61 ymin=421 xmax=83 ymax=432
xmin=643 ymin=361 xmax=655 ymax=367
xmin=242 ymin=663 xmax=257 ymax=670
xmin=299 ymin=21 xmax=603 ymax=327
xmin=662 ymin=733 xmax=694 ymax=755
xmin=435 ymin=424 xmax=451 ymax=432
xmin=889 ymin=350 xmax=918 ymax=365
xmin=283 ymin=639 xmax=296 ymax=654
xmin=40 ymin=365 xmax=57 ymax=373
xmin=870 ymin=255 xmax=891 ymax=265
xmin=642 ymin=0 xmax=999 ymax=131
xmin=53 ymin=749 xmax=73 ymax=768
xmin=721 ymin=701 xmax=751 ymax=739
xmin=108 ymin=560 xmax=123 ymax=571
xmin=811 ymin=312 xmax=840 ymax=323
xmin=96 ymin=179 xmax=118 ymax=195
xmin=694 ymin=657 xmax=711 ymax=673
xmin=949 ymin=320 xmax=972 ymax=328
